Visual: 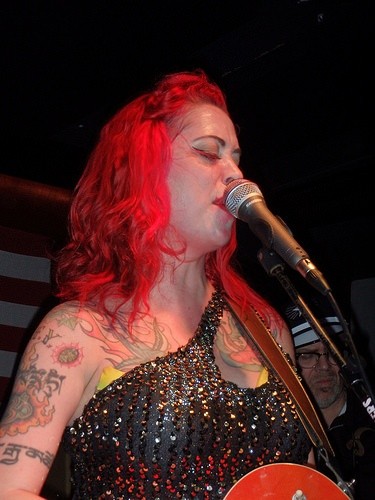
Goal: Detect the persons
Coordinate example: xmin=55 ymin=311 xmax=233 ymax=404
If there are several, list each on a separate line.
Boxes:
xmin=279 ymin=303 xmax=374 ymax=500
xmin=0 ymin=70 xmax=317 ymax=500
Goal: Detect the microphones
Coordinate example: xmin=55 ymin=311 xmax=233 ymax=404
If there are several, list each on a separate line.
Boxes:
xmin=222 ymin=178 xmax=331 ymax=295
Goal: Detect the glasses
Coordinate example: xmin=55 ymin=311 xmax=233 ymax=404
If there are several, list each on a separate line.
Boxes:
xmin=298 ymin=349 xmax=337 ymax=370
